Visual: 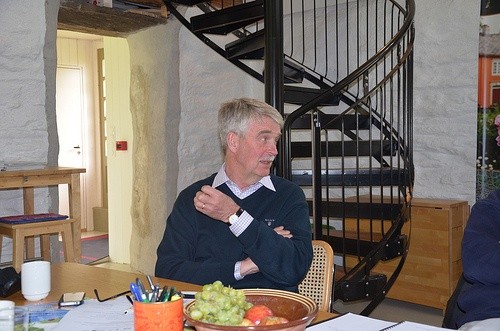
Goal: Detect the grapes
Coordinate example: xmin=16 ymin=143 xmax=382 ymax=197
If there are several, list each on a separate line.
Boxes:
xmin=190 ymin=280 xmax=254 ymax=325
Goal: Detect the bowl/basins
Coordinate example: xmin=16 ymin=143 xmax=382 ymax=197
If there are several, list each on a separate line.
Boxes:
xmin=183 ymin=288 xmax=319 ymax=331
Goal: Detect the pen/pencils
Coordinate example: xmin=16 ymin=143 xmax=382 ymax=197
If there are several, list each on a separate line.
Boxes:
xmin=150 ymin=284 xmax=160 ymax=302
xmin=160 ymin=285 xmax=182 ymax=303
xmin=147 ymin=275 xmax=156 ymax=291
xmin=130 ymin=282 xmax=142 ymax=303
xmin=136 ymin=278 xmax=149 ymax=302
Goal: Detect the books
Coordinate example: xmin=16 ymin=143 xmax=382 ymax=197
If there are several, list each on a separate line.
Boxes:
xmin=306 ymin=312 xmax=459 ymax=331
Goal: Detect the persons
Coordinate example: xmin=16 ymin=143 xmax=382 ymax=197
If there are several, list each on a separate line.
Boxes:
xmin=154 ymin=97 xmax=314 ymax=294
xmin=441 ymin=188 xmax=500 ymax=330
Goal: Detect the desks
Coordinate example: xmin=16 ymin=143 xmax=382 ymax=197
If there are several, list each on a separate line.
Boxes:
xmin=0 ymin=167 xmax=86 ymax=264
xmin=0 ymin=261 xmax=500 ymax=331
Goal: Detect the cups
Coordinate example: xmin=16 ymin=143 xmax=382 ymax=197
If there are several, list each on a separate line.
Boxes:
xmin=21 ymin=261 xmax=50 ymax=301
xmin=0 ymin=306 xmax=30 ymax=331
xmin=0 ymin=300 xmax=14 ymax=331
xmin=133 ymin=298 xmax=184 ymax=331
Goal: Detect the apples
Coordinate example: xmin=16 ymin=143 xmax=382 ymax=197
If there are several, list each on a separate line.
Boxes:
xmin=260 ymin=317 xmax=289 ymax=325
xmin=244 ymin=305 xmax=273 ymax=325
xmin=238 ymin=319 xmax=252 ymax=326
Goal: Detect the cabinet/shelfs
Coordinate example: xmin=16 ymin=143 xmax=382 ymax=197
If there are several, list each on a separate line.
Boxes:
xmin=343 ymin=194 xmax=469 ymax=317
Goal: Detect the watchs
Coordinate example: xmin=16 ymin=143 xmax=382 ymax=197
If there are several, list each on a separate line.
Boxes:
xmin=226 ymin=208 xmax=244 ymax=226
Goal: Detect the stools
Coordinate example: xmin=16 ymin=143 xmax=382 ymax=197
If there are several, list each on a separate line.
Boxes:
xmin=0 ymin=212 xmax=78 ymax=274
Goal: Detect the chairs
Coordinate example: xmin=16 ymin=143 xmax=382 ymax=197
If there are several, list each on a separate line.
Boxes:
xmin=297 ymin=241 xmax=333 ymax=312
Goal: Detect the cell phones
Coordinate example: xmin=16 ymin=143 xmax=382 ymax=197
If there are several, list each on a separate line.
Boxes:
xmin=58 ymin=292 xmax=85 ymax=307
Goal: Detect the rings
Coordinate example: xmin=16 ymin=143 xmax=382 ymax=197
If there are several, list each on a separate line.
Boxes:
xmin=202 ymin=203 xmax=206 ymax=209
